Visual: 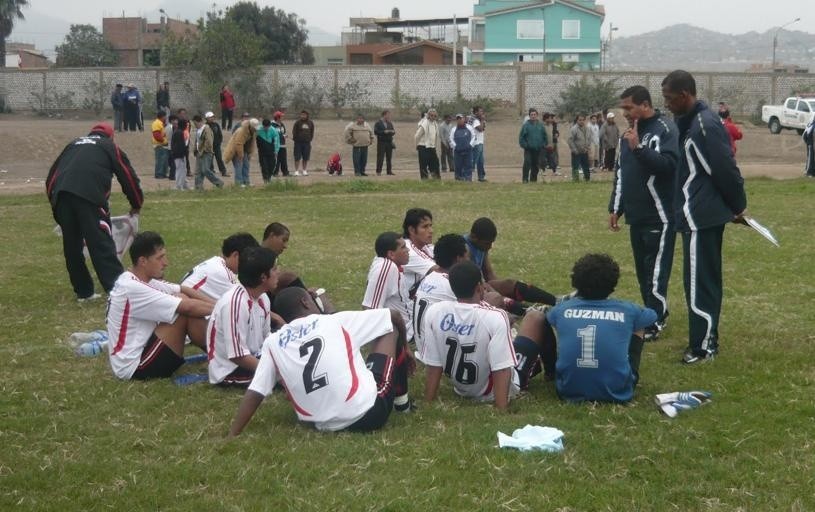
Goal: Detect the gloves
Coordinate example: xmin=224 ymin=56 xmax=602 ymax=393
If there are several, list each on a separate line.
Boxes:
xmin=655 ymin=391 xmax=712 ymax=418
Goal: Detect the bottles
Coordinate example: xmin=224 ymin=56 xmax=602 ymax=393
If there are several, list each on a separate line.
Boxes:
xmin=74 ymin=339 xmax=109 ymax=357
xmin=69 ymin=329 xmax=109 ymax=349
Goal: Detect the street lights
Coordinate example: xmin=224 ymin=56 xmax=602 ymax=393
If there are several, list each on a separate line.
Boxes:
xmin=158 ymin=8 xmax=171 ymax=19
xmin=540 ymin=6 xmax=549 ymax=60
xmin=771 ymin=16 xmax=802 ymax=104
xmin=603 ymin=27 xmax=619 ymax=71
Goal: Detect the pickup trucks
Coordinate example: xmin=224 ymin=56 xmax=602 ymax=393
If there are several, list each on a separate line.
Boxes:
xmin=759 ymin=95 xmax=815 ymax=135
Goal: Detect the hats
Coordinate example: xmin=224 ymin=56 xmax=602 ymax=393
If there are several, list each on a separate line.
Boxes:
xmin=91 ymin=123 xmax=113 ymax=137
xmin=273 ymin=111 xmax=284 ymax=116
xmin=250 ymin=119 xmax=260 ymax=130
xmin=205 ymin=111 xmax=214 ymax=119
xmin=607 ymin=112 xmax=614 ymax=119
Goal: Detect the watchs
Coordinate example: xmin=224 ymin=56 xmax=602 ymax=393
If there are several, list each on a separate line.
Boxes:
xmin=632 ymin=143 xmax=644 ymax=154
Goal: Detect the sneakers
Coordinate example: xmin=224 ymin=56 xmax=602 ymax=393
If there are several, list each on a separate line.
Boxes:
xmin=295 ymin=170 xmax=308 ymax=176
xmin=683 ymin=350 xmax=721 ymax=363
xmin=526 ymin=303 xmax=547 ymax=314
xmin=643 ymin=321 xmax=667 ymax=340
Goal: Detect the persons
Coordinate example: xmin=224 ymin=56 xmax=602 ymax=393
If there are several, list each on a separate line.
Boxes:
xmin=226 ymin=287 xmax=416 ymax=440
xmin=180 ymin=221 xmax=334 ymax=390
xmin=106 ymin=232 xmax=218 ymax=382
xmin=661 ymin=69 xmax=752 ymax=365
xmin=292 ymin=110 xmax=314 ymax=176
xmin=374 ymin=110 xmax=396 ymax=176
xmin=111 ymin=81 xmax=292 ymax=192
xmin=719 ymin=101 xmax=726 ymax=123
xmin=546 ymin=254 xmax=657 ymax=404
xmin=45 ymin=120 xmax=144 ymax=303
xmin=518 ymin=108 xmax=620 ymax=184
xmin=327 ymin=152 xmax=343 ymax=177
xmin=344 ymin=114 xmax=374 ymax=176
xmin=414 ymin=105 xmax=488 ymax=182
xmin=607 ymin=85 xmax=681 ymax=343
xmin=719 ymin=108 xmax=743 ymax=157
xmin=802 ymin=117 xmax=815 ymax=177
xmin=362 ymin=209 xmax=578 ymax=415
